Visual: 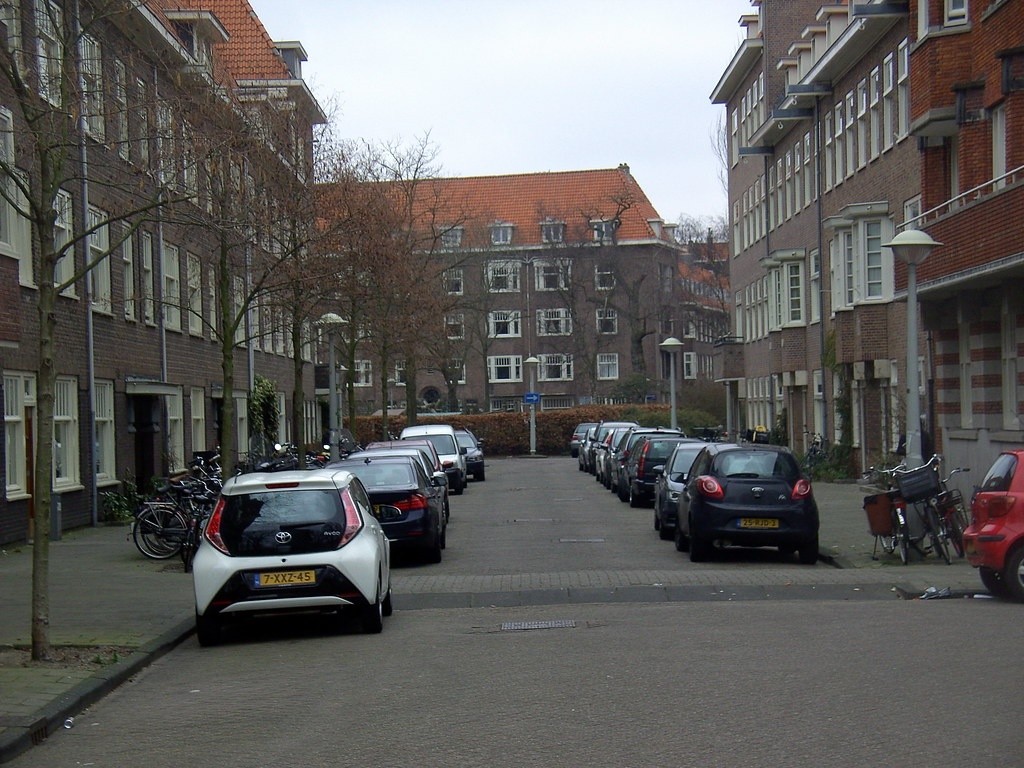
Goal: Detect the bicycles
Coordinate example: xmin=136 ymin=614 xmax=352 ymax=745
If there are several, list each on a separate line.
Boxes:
xmin=801 ymin=424 xmax=831 ymax=480
xmin=862 ymin=452 xmax=969 ymax=564
xmin=126 ymin=431 xmax=398 ymax=575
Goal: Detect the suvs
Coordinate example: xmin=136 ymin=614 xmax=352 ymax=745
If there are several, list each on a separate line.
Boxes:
xmin=588 ymin=420 xmax=689 ymax=490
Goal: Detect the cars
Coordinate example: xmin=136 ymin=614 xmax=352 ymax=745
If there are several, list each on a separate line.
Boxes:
xmin=962 ymin=449 xmax=1024 ymax=601
xmin=617 ymin=437 xmax=708 ymax=508
xmin=578 ymin=427 xmax=597 ymax=471
xmin=652 ymin=440 xmax=746 ymax=541
xmin=324 ymin=454 xmax=448 ymax=564
xmin=571 ymin=423 xmax=600 ymax=458
xmin=670 ymin=440 xmax=819 ymax=567
xmin=346 ymin=439 xmax=450 ymax=525
xmin=192 ymin=469 xmax=401 ymax=648
xmin=454 ymin=426 xmax=487 ymax=483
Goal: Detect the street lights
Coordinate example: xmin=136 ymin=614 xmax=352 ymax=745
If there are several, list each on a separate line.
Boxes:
xmin=313 ymin=311 xmax=351 ymax=463
xmin=523 ymin=356 xmax=541 ymax=456
xmin=879 ymin=230 xmax=943 ymax=563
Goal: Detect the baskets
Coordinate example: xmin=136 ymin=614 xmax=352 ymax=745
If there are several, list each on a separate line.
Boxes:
xmin=897 ymin=465 xmax=939 ymax=504
xmin=948 ymin=489 xmax=963 ymax=504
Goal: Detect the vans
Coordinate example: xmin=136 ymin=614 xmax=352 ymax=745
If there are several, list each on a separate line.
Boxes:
xmin=398 ymin=423 xmax=468 ymax=496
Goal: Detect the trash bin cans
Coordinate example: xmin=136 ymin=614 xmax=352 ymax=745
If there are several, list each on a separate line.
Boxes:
xmin=693 ymin=426 xmax=720 ymax=437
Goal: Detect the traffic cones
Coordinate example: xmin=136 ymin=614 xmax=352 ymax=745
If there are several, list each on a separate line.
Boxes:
xmin=657 ymin=338 xmax=685 ymax=430
